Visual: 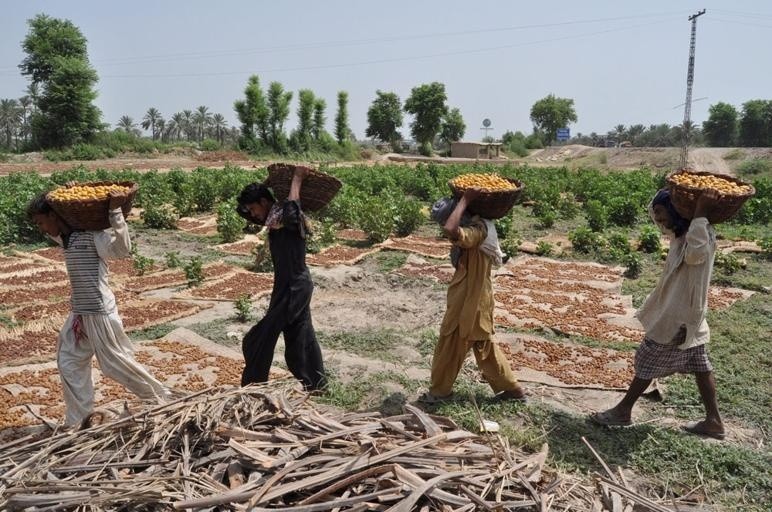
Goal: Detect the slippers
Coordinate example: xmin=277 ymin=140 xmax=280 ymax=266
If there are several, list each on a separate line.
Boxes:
xmin=588 ymin=411 xmax=634 ymax=426
xmin=489 ymin=393 xmax=528 ymax=403
xmin=684 ymin=420 xmax=727 ymax=441
xmin=417 ymin=391 xmax=456 ymax=404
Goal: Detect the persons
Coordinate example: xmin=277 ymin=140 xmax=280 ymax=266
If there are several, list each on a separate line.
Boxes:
xmin=27 ymin=180 xmax=169 ymax=425
xmin=419 ymin=186 xmax=524 ymax=404
xmin=236 ymin=163 xmax=327 ymax=395
xmin=589 ymin=167 xmax=725 ymax=440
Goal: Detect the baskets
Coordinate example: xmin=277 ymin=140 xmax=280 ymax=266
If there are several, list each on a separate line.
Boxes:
xmin=447 ymin=173 xmax=526 ymax=220
xmin=44 ymin=179 xmax=140 ymax=232
xmin=664 ymin=168 xmax=757 ymax=225
xmin=266 ymin=162 xmax=343 ymax=213
